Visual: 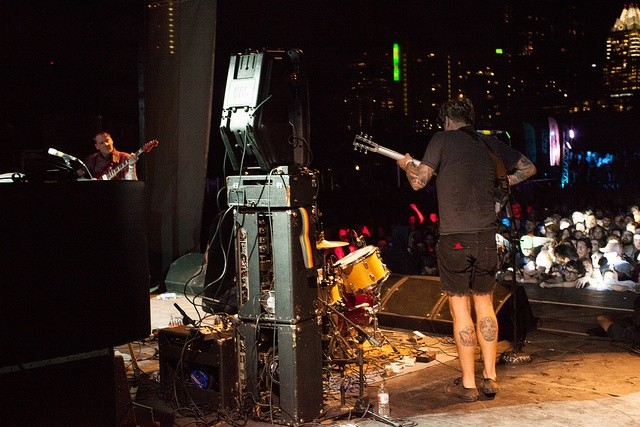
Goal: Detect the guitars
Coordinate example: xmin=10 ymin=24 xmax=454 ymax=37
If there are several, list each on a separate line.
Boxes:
xmin=98 ymin=138 xmax=159 ymax=181
xmin=352 ymin=131 xmax=510 ymax=213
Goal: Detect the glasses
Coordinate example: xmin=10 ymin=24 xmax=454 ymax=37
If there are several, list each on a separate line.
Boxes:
xmin=565 ymin=269 xmax=579 ymax=275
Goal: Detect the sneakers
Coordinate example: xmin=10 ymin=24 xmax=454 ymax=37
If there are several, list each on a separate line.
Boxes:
xmin=444 ymin=376 xmax=479 ymax=401
xmin=476 ymin=377 xmax=499 ymax=397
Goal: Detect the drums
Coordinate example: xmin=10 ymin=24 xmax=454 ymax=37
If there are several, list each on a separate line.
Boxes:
xmin=315 ymin=267 xmax=341 ymax=314
xmin=333 ymin=243 xmax=390 ymax=295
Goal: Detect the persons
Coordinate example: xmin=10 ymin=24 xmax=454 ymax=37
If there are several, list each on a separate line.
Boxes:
xmin=77 ymin=131 xmax=139 ymax=180
xmin=375 ymin=205 xmax=640 ymax=291
xmin=398 ymin=96 xmax=537 ymax=401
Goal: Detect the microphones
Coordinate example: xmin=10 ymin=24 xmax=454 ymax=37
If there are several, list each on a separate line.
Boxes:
xmin=48 ymin=148 xmax=94 ymax=180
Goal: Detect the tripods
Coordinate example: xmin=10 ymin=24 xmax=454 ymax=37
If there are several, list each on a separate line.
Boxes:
xmin=317 ymin=297 xmax=402 ymax=427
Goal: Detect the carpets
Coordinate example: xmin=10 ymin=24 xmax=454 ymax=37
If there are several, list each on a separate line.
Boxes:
xmin=135 ymin=326 xmax=481 ymax=391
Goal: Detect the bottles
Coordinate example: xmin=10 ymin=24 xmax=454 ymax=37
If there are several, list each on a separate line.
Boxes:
xmin=377 ymin=383 xmax=390 ymax=418
xmin=158 ymin=293 xmax=176 ymax=300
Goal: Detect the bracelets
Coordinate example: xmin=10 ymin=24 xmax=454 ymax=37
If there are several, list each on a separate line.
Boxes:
xmin=406 ymin=160 xmax=413 ymax=165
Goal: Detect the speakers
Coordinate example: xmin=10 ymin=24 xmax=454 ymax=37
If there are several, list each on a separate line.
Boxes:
xmin=378 ymin=273 xmax=406 ymax=302
xmin=377 ymin=274 xmax=450 ymax=333
xmin=1 ymin=356 xmax=139 ymax=427
xmin=432 ymin=279 xmax=536 ymax=344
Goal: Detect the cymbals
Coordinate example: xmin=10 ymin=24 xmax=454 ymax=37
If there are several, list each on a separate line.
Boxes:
xmin=315 ymin=240 xmax=351 ymax=248
